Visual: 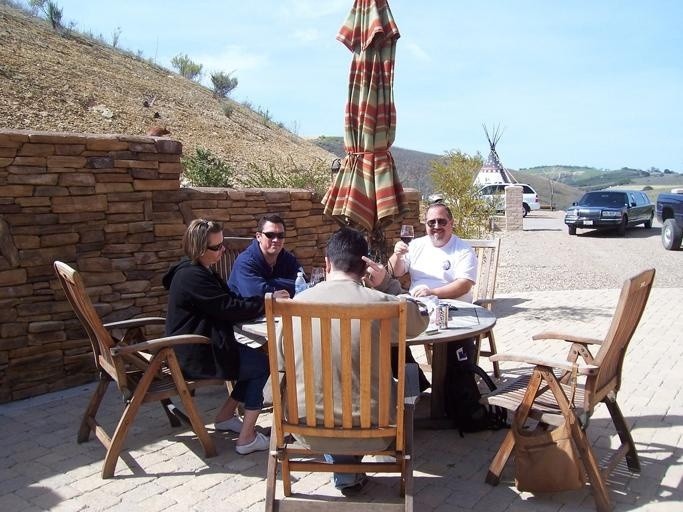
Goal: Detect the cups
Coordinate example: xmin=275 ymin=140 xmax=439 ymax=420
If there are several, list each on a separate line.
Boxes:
xmin=308 ymin=267 xmax=325 ymax=288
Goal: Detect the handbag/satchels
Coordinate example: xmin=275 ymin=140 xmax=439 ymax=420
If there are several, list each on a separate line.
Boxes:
xmin=513 ymin=422 xmax=582 ymax=492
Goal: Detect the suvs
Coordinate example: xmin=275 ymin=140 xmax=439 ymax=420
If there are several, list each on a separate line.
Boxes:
xmin=458 ymin=182 xmax=542 ymax=217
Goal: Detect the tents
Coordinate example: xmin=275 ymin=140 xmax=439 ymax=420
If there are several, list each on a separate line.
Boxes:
xmin=472 ymin=119 xmax=511 ymax=186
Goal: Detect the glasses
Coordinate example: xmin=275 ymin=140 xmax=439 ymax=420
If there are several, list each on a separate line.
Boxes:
xmin=262 ymin=232 xmax=284 ymax=238
xmin=427 ymin=219 xmax=447 ymax=227
xmin=207 ymin=242 xmax=223 ymax=250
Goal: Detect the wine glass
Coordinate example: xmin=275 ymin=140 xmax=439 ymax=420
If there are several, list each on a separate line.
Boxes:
xmin=400 ymin=225 xmax=414 ymax=244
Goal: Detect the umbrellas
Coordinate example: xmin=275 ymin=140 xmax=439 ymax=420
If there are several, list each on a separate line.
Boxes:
xmin=320 ymin=0 xmax=409 ymax=257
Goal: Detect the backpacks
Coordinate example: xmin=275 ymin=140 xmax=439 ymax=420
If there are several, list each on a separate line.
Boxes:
xmin=448 ymin=342 xmax=511 ymax=437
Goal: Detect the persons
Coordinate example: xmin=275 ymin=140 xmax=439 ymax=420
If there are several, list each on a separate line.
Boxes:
xmin=225 ymin=213 xmax=308 ymax=302
xmin=386 ymin=203 xmax=477 ymax=421
xmin=280 ymin=227 xmax=428 ymax=491
xmin=160 ymin=218 xmax=270 ymax=455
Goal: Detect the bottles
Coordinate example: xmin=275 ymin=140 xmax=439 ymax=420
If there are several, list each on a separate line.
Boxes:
xmin=294 ymin=271 xmax=306 ymax=293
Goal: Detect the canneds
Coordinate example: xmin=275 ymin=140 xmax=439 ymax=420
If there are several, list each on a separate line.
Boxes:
xmin=435 ymin=304 xmax=448 ymax=330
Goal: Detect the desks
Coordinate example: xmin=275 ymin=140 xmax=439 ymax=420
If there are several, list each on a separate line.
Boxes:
xmin=236 ymin=296 xmax=498 ymax=432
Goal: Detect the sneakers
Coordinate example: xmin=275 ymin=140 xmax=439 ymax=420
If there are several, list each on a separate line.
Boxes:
xmin=341 ymin=477 xmax=375 ymax=497
xmin=236 ymin=432 xmax=269 ymax=455
xmin=214 ymin=416 xmax=244 ymax=432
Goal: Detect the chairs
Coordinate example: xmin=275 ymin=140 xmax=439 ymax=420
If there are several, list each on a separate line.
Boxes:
xmin=52 ymin=259 xmax=225 ymax=479
xmin=484 ymin=268 xmax=657 ymax=512
xmin=424 ymin=237 xmax=502 ymax=380
xmin=209 ymin=237 xmax=274 ymax=416
xmin=262 ymin=291 xmax=422 ymax=511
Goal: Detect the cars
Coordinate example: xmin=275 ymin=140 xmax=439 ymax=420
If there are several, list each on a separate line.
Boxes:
xmin=422 ymin=193 xmax=451 ymax=204
xmin=656 ymin=188 xmax=683 ymax=250
xmin=565 ymin=186 xmax=655 ymax=238
